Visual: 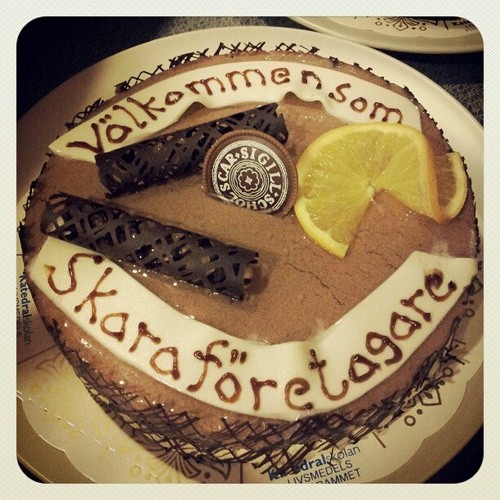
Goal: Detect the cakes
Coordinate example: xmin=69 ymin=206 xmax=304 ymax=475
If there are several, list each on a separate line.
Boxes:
xmin=16 ymin=42 xmax=481 ymax=474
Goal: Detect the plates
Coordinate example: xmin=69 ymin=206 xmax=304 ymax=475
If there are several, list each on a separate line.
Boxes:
xmin=279 ymin=15 xmax=483 ymax=58
xmin=17 ymin=25 xmax=484 ymax=482
